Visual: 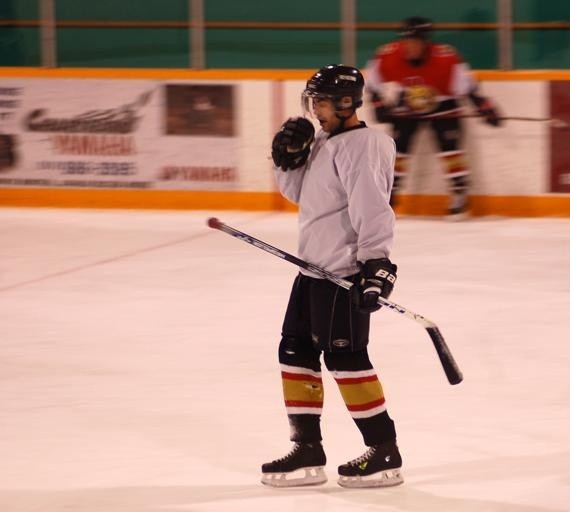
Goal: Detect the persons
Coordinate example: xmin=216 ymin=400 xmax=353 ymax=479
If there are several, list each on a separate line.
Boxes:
xmin=260 ymin=62 xmax=403 ymax=478
xmin=360 ymin=15 xmax=502 ymax=218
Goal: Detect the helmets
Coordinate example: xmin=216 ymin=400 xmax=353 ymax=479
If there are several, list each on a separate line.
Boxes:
xmin=305 ymin=63 xmax=366 ymax=111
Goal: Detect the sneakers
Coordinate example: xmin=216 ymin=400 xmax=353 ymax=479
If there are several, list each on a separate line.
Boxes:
xmin=338 ymin=442 xmax=403 ymax=477
xmin=261 ymin=439 xmax=327 ymax=474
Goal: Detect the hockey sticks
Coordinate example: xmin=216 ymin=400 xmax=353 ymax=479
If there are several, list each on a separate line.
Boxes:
xmin=422 ymin=112 xmax=569 ymax=129
xmin=207 ymin=218 xmax=464 ymax=386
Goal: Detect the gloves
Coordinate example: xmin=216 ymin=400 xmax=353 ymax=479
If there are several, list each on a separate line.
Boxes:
xmin=270 ymin=116 xmax=316 ymax=171
xmin=351 ymin=257 xmax=399 ymax=313
